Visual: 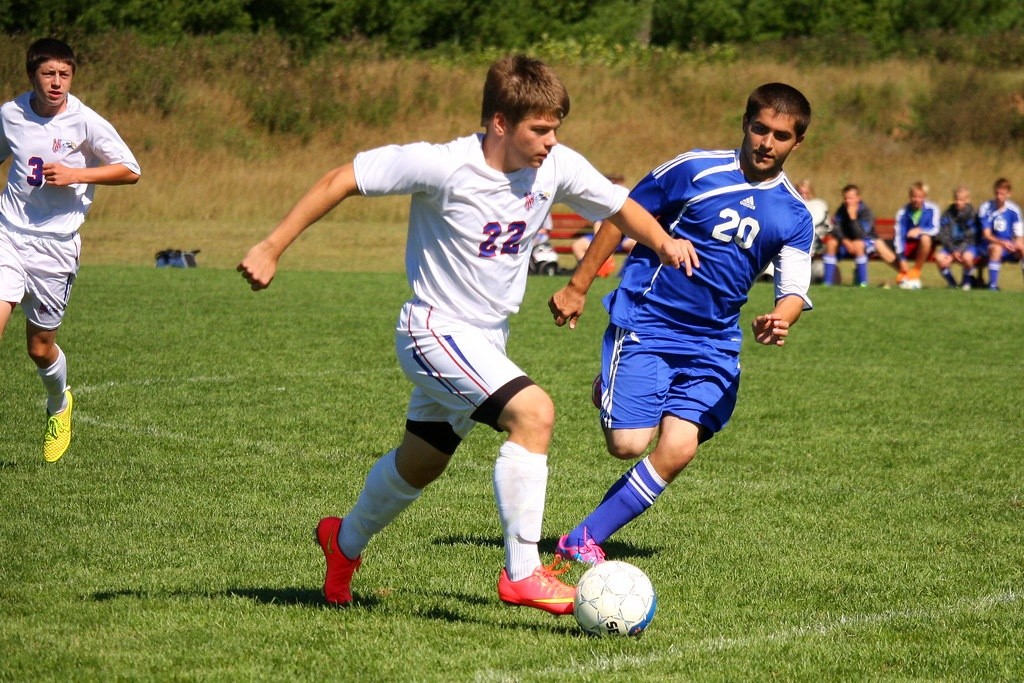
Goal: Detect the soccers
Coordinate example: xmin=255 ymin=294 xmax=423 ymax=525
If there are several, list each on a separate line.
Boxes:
xmin=899 ymin=276 xmax=921 ymax=290
xmin=574 ymin=561 xmax=655 ymax=637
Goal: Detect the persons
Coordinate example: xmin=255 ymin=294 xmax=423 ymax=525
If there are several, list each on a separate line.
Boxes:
xmin=1 ymin=36 xmax=142 ymax=464
xmin=821 ymin=184 xmax=879 ymax=291
xmin=931 ymin=185 xmax=987 ymax=288
xmin=978 ymin=179 xmax=1024 ymax=293
xmin=873 ymin=183 xmax=943 ymax=294
xmin=528 ymin=212 xmax=554 ymax=274
xmin=550 ymin=83 xmax=816 ymax=571
xmin=574 ymin=175 xmax=640 ymax=271
xmin=237 ymin=49 xmax=704 ymax=613
xmin=758 ymin=180 xmax=833 ymax=283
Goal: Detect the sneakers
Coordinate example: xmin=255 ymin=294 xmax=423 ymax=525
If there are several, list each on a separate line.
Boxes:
xmin=553 ymin=533 xmax=606 ymax=569
xmin=42 ymin=390 xmax=74 ymax=463
xmin=314 ymin=516 xmax=363 ymax=609
xmin=497 ymin=565 xmax=578 ymax=615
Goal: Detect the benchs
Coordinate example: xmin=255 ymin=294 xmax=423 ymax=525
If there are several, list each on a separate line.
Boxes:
xmin=813 ymin=217 xmax=1021 ymax=266
xmin=548 ymin=213 xmax=598 ymax=254
